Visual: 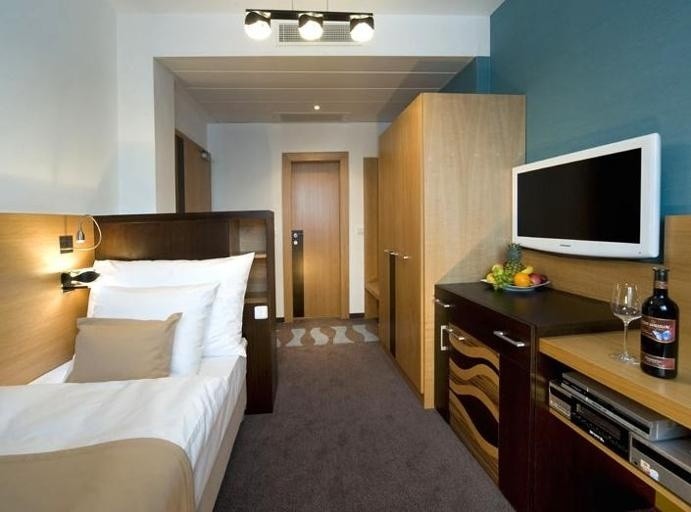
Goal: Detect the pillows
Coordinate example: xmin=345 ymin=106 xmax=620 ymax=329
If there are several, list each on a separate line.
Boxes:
xmin=61 ymin=311 xmax=184 ymax=383
xmin=86 ymin=280 xmax=222 ymax=378
xmin=85 ymin=252 xmax=255 ymax=361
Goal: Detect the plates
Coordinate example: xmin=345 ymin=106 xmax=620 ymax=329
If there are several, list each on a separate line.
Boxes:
xmin=480 ymin=278 xmax=551 ymax=294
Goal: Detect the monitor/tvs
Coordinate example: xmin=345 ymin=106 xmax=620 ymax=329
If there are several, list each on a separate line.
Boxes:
xmin=511 ymin=133 xmax=661 ymax=258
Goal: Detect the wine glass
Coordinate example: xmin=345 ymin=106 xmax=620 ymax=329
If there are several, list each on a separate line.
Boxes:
xmin=609 ymin=279 xmax=644 ymax=366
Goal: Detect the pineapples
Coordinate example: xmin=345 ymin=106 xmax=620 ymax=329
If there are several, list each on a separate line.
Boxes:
xmin=504 ymin=260 xmax=521 ymax=275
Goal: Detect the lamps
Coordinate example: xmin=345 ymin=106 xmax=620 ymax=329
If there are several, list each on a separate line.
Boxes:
xmin=60 ymin=210 xmax=103 ymax=256
xmin=242 ymin=0 xmax=376 ymax=47
xmin=59 ymin=268 xmax=99 ymax=292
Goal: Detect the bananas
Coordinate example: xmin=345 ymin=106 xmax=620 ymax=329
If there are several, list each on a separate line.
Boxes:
xmin=521 ymin=266 xmax=534 ymax=275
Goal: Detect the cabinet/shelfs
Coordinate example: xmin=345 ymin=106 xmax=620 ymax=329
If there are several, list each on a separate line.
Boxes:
xmin=435 ymin=278 xmax=656 ymax=511
xmin=377 ymin=92 xmax=527 ymax=408
xmin=92 ymin=212 xmax=277 ymax=414
xmin=537 ymin=328 xmax=691 ymax=511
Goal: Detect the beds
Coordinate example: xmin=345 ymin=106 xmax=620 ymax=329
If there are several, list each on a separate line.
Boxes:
xmin=0 ymin=252 xmax=256 ymax=510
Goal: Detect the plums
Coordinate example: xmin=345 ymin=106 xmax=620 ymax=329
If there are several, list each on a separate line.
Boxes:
xmin=540 ymin=275 xmax=547 ymax=283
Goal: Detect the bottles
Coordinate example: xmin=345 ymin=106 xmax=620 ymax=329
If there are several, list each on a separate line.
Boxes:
xmin=641 ymin=265 xmax=680 ymax=380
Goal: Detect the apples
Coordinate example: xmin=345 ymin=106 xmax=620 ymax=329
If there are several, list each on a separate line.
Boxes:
xmin=529 ymin=274 xmax=541 ymax=286
